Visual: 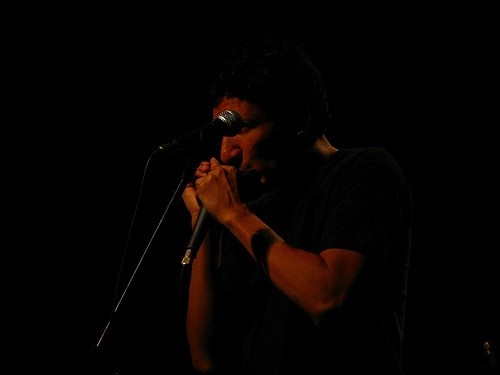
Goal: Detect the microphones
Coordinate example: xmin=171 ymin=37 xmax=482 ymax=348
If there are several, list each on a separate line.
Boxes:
xmin=182 ymin=205 xmax=213 ymax=265
xmin=158 ymin=109 xmax=243 ymax=151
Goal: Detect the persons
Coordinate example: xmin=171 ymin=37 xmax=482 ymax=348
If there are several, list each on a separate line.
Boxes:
xmin=180 ymin=39 xmax=413 ymax=375
